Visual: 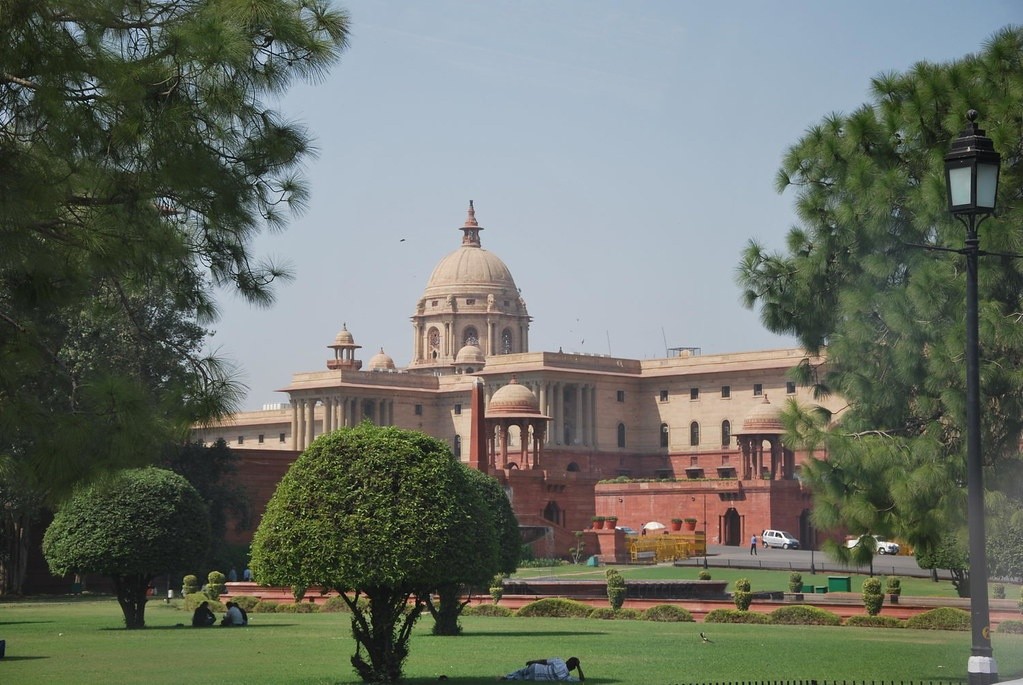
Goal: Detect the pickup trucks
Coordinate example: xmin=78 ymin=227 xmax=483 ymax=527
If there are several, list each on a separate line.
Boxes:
xmin=847 ymin=534 xmax=900 ymax=556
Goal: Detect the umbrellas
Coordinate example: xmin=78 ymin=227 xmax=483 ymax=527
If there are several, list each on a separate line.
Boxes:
xmin=644 ymin=521 xmax=666 ymax=530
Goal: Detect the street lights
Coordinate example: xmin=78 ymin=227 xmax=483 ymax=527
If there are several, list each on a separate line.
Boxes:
xmin=942 ymin=108 xmax=1003 ymax=685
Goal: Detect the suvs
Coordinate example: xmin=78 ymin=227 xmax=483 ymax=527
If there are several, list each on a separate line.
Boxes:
xmin=761 ymin=530 xmax=800 ymax=550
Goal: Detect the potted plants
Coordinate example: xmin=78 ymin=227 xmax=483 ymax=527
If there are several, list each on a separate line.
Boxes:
xmin=605 ymin=516 xmax=617 ymax=529
xmin=592 ymin=516 xmax=605 ymax=529
xmin=685 ymin=519 xmax=697 ymax=531
xmin=671 ymin=519 xmax=682 ymax=530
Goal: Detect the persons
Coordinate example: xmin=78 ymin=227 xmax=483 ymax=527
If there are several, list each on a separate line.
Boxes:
xmin=146 ymin=566 xmax=253 ymax=627
xmin=750 ymin=535 xmax=757 ymax=555
xmin=498 ymin=657 xmax=585 ymax=683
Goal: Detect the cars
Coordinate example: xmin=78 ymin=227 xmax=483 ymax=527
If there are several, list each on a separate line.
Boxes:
xmin=615 ymin=526 xmax=639 ymax=536
